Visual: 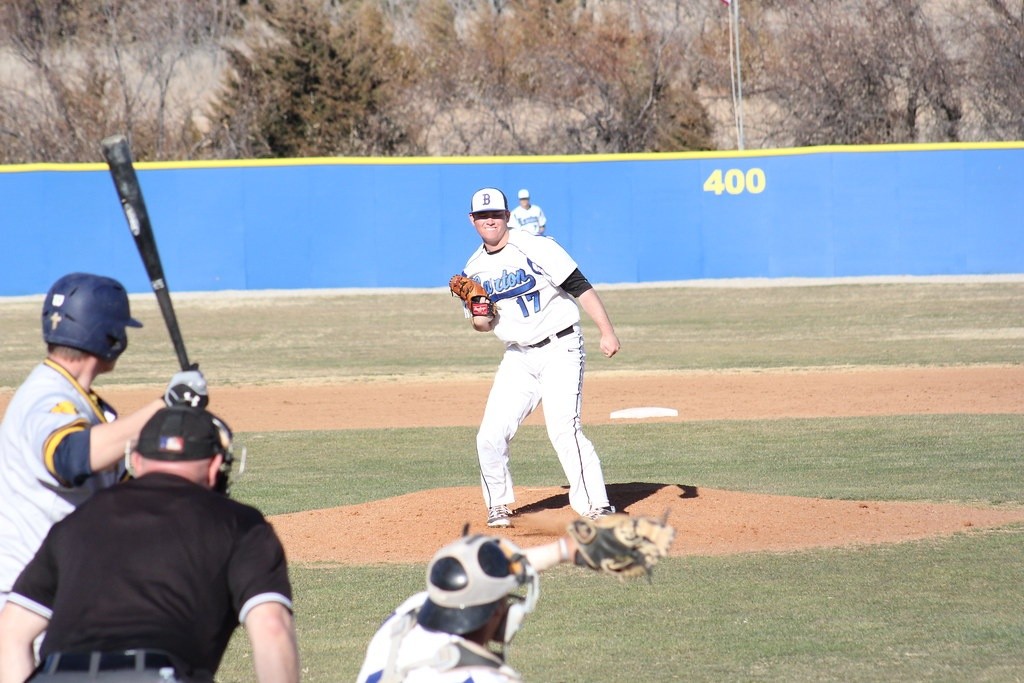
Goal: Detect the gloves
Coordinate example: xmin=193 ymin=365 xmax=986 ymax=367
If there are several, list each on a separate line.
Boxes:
xmin=160 ymin=361 xmax=209 ymax=410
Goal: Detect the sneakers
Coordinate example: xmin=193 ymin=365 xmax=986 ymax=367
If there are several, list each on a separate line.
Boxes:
xmin=485 ymin=503 xmax=513 ymax=527
xmin=583 ymin=505 xmax=616 ymax=520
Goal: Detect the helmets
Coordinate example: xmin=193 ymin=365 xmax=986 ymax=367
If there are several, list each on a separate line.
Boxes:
xmin=42 ymin=273 xmax=143 ymax=361
xmin=416 ymin=532 xmax=519 ymax=635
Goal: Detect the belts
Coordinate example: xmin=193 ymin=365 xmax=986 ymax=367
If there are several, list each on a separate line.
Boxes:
xmin=530 ymin=325 xmax=574 ymax=348
xmin=42 ymin=653 xmax=181 ymax=681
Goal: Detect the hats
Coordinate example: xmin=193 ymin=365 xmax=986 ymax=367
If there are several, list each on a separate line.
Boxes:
xmin=518 ymin=189 xmax=530 ymax=199
xmin=470 ymin=187 xmax=508 ymax=214
xmin=135 ymin=408 xmax=225 ymax=461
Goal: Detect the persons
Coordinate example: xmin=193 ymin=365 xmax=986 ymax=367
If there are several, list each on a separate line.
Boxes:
xmin=507 ymin=189 xmax=547 ymax=234
xmin=0 ymin=273 xmax=208 ymax=663
xmin=354 ymin=506 xmax=676 ymax=683
xmin=449 ymin=187 xmax=621 ymax=527
xmin=0 ymin=402 xmax=300 ymax=683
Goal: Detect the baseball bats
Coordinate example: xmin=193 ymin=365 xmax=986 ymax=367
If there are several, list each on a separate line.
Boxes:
xmin=100 ymin=132 xmax=201 ymax=373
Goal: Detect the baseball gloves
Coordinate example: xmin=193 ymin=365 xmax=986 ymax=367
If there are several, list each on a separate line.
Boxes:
xmin=565 ymin=515 xmax=676 ymax=577
xmin=450 ymin=274 xmax=497 ymax=322
